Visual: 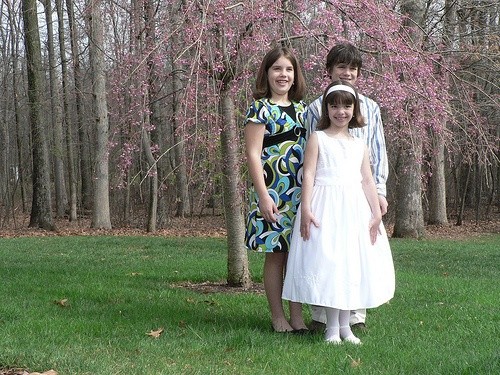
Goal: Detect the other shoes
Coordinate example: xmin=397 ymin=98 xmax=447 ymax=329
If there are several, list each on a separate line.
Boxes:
xmin=340 ymin=326 xmax=360 ymax=344
xmin=351 ymin=323 xmax=368 ymax=331
xmin=289 ymin=318 xmax=309 ymax=332
xmin=309 ymin=320 xmax=326 ymax=334
xmin=272 ymin=319 xmax=294 ymax=333
xmin=324 ymin=326 xmax=341 ymax=344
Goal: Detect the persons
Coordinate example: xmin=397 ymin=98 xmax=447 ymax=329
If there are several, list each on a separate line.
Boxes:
xmin=305 ymin=43 xmax=391 ymax=339
xmin=282 ymin=83 xmax=396 ymax=346
xmin=242 ymin=46 xmax=309 ymax=335
